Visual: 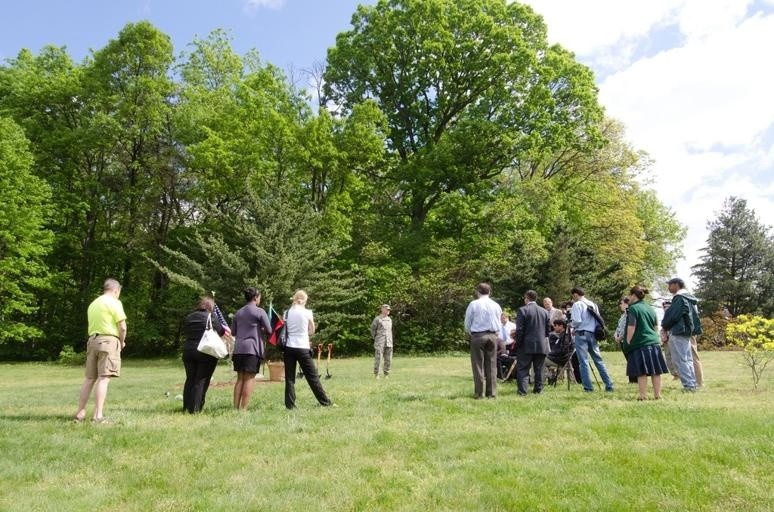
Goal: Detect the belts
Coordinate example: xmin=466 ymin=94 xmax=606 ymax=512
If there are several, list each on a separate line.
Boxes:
xmin=471 ymin=332 xmax=496 ymax=334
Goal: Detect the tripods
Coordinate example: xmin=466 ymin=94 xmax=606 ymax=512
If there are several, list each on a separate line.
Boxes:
xmin=554 ymin=320 xmax=602 ymax=391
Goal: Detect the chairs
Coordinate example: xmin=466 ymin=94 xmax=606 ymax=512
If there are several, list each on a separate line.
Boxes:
xmin=548 ymin=349 xmax=577 ymax=390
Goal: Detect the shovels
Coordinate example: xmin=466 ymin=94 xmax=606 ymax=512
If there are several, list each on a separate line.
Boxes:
xmin=297 ymin=366 xmax=303 ymax=379
xmin=325 ymin=344 xmax=333 ymax=379
xmin=317 ymin=344 xmax=322 ymax=378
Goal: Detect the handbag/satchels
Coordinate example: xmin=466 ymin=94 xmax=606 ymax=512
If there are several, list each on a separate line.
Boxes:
xmin=197 ymin=313 xmax=229 ymax=360
xmin=595 ymin=326 xmax=605 ymax=341
xmin=277 ymin=310 xmax=288 ymax=351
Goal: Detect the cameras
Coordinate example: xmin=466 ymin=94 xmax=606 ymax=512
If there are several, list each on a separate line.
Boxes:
xmin=563 ymin=310 xmax=572 ymax=320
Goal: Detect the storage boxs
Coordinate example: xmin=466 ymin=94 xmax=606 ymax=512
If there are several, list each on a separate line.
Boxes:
xmin=268 ymin=362 xmax=285 ymax=382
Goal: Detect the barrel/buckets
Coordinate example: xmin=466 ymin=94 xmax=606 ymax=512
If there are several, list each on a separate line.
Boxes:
xmin=267 ymin=361 xmax=286 ymax=382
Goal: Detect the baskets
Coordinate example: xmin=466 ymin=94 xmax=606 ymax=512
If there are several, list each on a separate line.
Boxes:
xmin=267 ymin=360 xmax=285 ymax=381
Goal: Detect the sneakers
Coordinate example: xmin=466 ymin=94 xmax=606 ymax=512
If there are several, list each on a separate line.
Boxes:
xmin=73 ymin=417 xmax=86 ymax=424
xmin=91 ymin=418 xmax=115 ymax=425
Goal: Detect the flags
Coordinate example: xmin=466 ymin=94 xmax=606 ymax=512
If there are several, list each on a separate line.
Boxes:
xmin=267 ymin=305 xmax=284 ymax=346
xmin=212 ymin=303 xmax=232 ymax=338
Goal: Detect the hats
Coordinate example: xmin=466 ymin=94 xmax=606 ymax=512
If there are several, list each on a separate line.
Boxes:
xmin=381 ymin=304 xmax=391 ymax=310
xmin=666 ymin=278 xmax=684 ymax=283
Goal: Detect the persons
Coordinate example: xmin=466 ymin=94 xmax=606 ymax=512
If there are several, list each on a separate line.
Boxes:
xmin=73 ymin=279 xmax=127 ymax=424
xmin=463 ymin=283 xmax=503 ymax=399
xmin=282 ymin=289 xmax=338 ymax=410
xmin=496 ymin=289 xmax=581 ymax=395
xmin=568 ymin=287 xmax=613 ymax=392
xmin=180 ymin=296 xmax=225 ymax=416
xmin=370 ymin=304 xmax=394 ymax=379
xmin=230 ymin=288 xmax=272 ymax=412
xmin=614 ymin=278 xmax=705 ymax=402
